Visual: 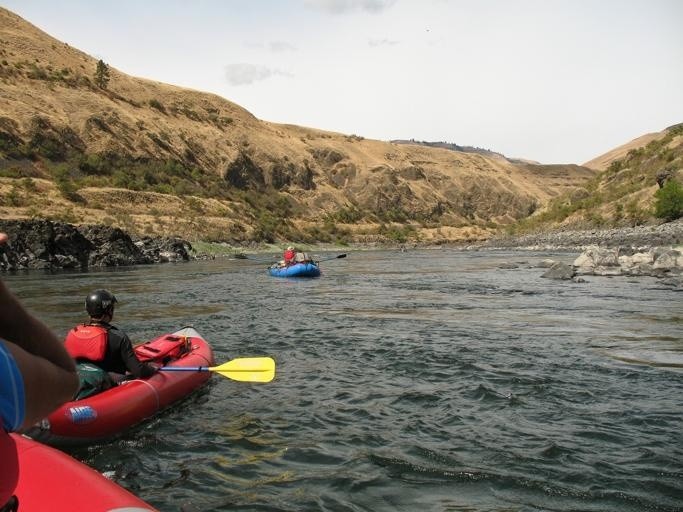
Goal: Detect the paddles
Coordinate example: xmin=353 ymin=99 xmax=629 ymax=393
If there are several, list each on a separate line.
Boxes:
xmin=314 ymin=254 xmax=348 ymax=263
xmin=150 ymin=357 xmax=275 ymax=383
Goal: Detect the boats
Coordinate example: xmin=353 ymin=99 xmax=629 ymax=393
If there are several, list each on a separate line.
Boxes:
xmin=0 ymin=430 xmax=160 ymax=512
xmin=32 ymin=326 xmax=214 ymax=440
xmin=267 ymin=261 xmax=320 ymax=277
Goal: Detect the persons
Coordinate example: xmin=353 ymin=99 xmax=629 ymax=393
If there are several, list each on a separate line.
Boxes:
xmin=290 ymin=250 xmax=304 ymax=263
xmin=0 ymin=232 xmax=78 ymax=511
xmin=284 ymin=245 xmax=295 ymax=262
xmin=63 ymin=288 xmax=162 ymax=402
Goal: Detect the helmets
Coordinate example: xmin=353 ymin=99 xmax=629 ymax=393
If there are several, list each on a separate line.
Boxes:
xmin=85 ymin=289 xmax=118 ymax=316
